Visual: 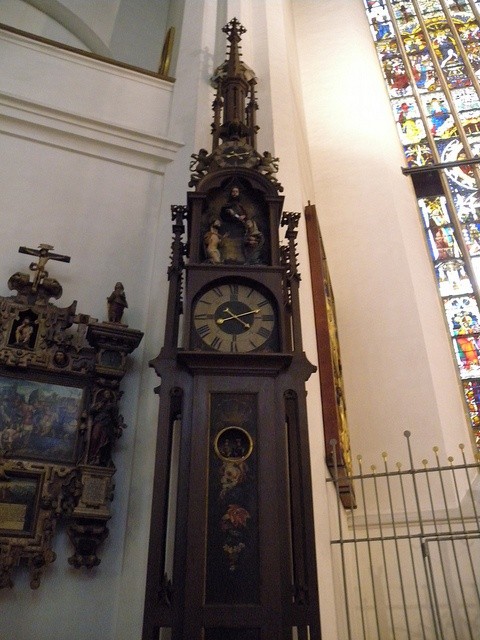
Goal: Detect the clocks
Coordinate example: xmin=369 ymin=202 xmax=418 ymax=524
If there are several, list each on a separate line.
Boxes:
xmin=192 ymin=282 xmax=276 ymax=354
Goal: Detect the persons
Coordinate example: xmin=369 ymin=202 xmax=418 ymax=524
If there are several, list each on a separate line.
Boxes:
xmin=0 ymin=390 xmax=78 ymax=458
xmin=232 ymin=438 xmax=245 ymax=458
xmin=243 ymin=219 xmax=264 ymax=263
xmin=55 ymin=351 xmax=65 ymax=365
xmin=202 ymin=218 xmax=223 ymax=263
xmin=221 ymin=187 xmax=247 ymax=264
xmin=107 ymin=282 xmax=128 ymax=324
xmin=221 ymin=438 xmax=231 ymax=458
xmin=15 ymin=318 xmax=33 ymax=345
